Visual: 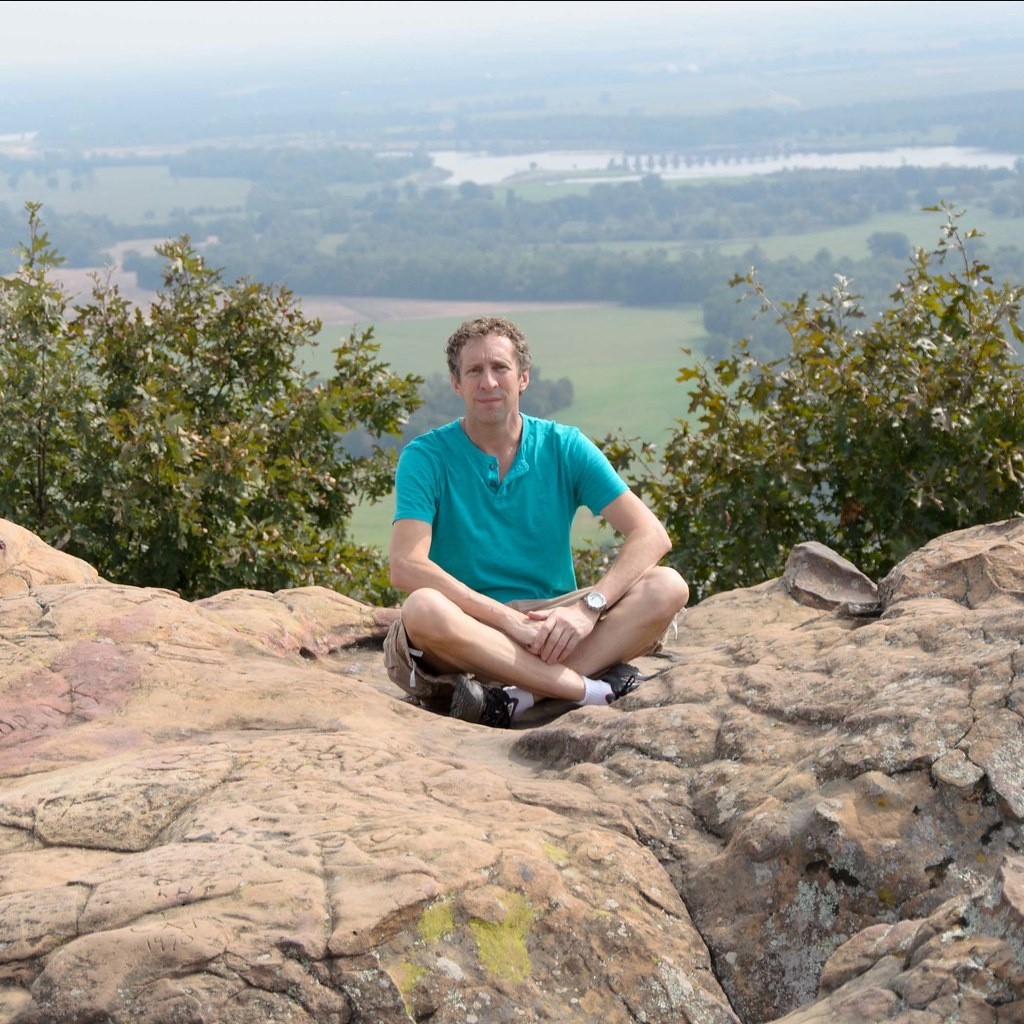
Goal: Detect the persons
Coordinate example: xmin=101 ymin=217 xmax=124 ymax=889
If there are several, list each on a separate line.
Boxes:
xmin=382 ymin=316 xmax=690 ymax=728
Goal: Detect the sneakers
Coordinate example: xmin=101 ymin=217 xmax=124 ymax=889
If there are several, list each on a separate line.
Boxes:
xmin=450 ymin=674 xmax=517 ymax=727
xmin=595 ymin=662 xmax=645 ymax=700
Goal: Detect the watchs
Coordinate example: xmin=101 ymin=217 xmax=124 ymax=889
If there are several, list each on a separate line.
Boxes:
xmin=582 ymin=591 xmax=606 ymax=612
